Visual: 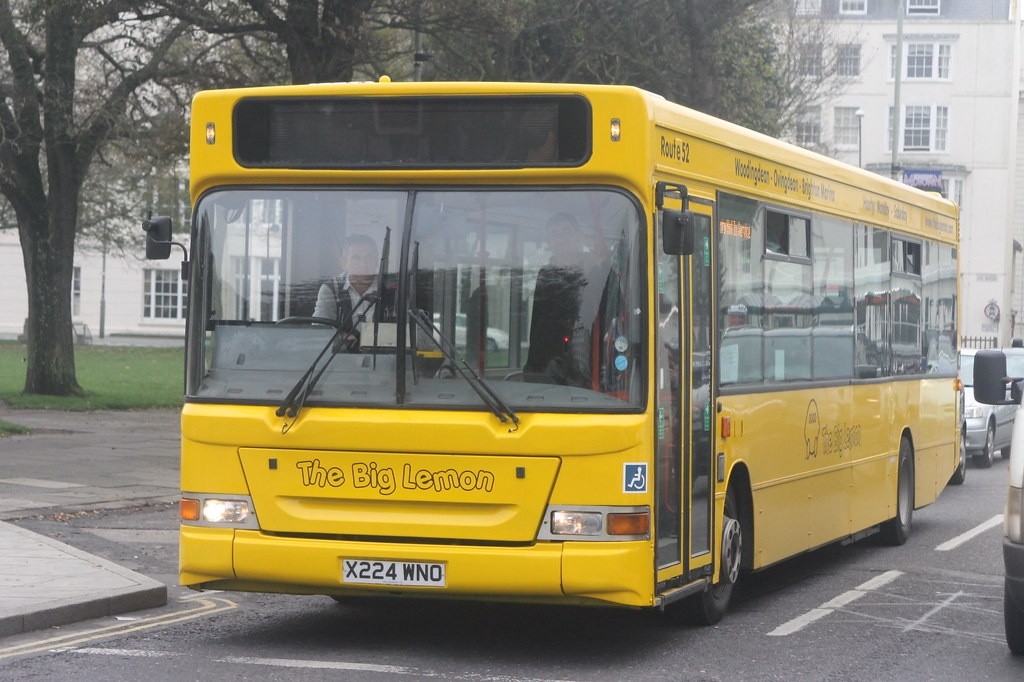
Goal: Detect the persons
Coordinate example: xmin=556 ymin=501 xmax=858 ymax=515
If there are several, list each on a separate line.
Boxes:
xmin=310 ymin=233 xmax=413 ymax=352
xmin=524 ymin=209 xmax=628 ymax=394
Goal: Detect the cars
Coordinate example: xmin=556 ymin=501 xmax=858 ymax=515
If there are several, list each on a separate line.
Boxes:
xmin=962 ymin=347 xmax=1023 ymax=464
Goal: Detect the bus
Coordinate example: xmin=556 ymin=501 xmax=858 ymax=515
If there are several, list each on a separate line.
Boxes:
xmin=142 ymin=80 xmax=963 ymax=624
